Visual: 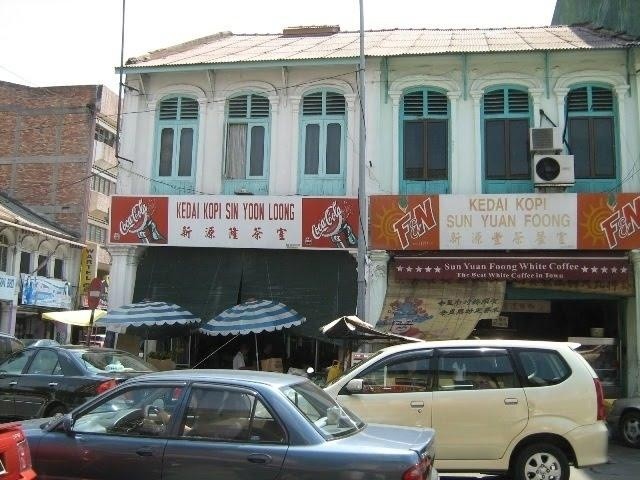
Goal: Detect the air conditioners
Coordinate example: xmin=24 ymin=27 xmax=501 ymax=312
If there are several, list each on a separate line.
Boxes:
xmin=528 ymin=125 xmax=564 ymax=153
xmin=531 ymin=154 xmax=577 ymax=192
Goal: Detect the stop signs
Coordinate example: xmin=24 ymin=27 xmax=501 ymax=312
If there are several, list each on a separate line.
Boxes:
xmin=87 ymin=278 xmax=102 ymax=310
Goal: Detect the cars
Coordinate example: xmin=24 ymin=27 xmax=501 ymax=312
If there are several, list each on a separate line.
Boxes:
xmin=604 ymin=396 xmax=640 ymax=449
xmin=0 ymin=414 xmax=39 ymax=479
xmin=9 ymin=369 xmax=440 ymax=480
xmin=1 ymin=333 xmax=26 ymax=366
xmin=0 ymin=339 xmax=162 ymax=423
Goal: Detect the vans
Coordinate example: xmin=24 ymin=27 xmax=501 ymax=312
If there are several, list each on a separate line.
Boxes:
xmin=320 ymin=340 xmax=610 ymax=480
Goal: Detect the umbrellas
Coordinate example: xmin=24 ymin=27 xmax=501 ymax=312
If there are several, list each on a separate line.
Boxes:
xmin=95 ymin=297 xmax=201 ymax=363
xmin=200 ymin=298 xmax=307 ymax=371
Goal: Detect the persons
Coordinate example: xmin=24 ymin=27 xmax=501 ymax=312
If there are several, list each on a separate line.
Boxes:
xmin=452 ymin=357 xmax=471 ymax=385
xmin=232 ymin=341 xmax=249 ymax=370
xmin=139 ymin=388 xmax=222 ymax=437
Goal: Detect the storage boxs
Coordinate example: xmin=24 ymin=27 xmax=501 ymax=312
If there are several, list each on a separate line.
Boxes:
xmin=260 ymin=357 xmax=284 ymax=373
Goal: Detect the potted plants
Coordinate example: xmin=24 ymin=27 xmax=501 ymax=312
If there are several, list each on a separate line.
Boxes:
xmin=149 ymin=350 xmax=176 ymax=372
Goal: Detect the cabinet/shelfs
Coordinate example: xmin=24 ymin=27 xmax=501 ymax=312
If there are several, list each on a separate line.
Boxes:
xmin=568 ymin=335 xmax=618 ymax=387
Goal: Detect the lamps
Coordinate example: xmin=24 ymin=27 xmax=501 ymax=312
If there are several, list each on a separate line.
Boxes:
xmin=307 ymin=366 xmax=316 ymax=377
